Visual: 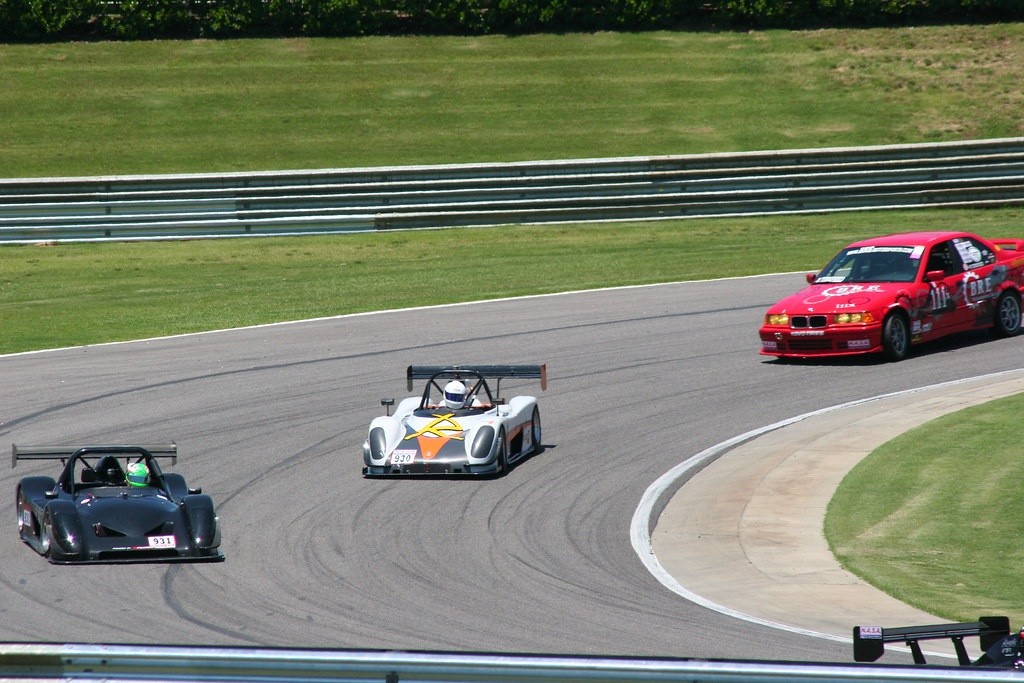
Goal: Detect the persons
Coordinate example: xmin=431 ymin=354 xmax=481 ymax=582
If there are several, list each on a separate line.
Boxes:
xmin=438 ymin=380 xmax=467 ymax=409
xmin=125 ymin=462 xmax=151 ymax=488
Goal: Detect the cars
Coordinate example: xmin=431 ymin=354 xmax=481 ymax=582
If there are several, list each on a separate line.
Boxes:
xmin=760 ymin=232 xmax=1022 ymax=362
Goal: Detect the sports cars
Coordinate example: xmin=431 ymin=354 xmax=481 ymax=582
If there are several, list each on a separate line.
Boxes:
xmin=10 ymin=441 xmax=225 ymax=565
xmin=361 ymin=362 xmax=548 ymax=480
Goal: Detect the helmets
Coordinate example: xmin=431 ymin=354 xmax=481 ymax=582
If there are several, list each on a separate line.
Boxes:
xmin=443 ymin=381 xmax=466 ymax=409
xmin=126 ymin=463 xmax=152 ymax=487
